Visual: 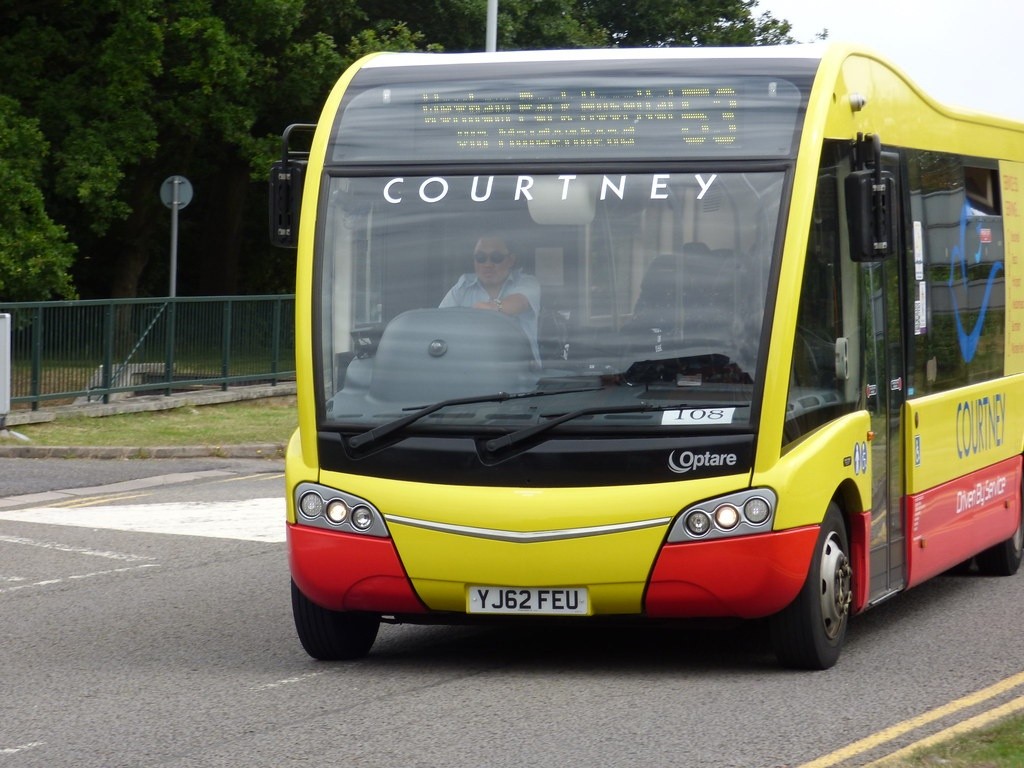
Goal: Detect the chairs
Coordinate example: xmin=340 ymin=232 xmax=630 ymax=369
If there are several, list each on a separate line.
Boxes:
xmin=628 ymin=243 xmax=754 ymax=340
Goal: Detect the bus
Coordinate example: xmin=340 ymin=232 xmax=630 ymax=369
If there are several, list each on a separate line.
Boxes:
xmin=266 ymin=48 xmax=1024 ymax=670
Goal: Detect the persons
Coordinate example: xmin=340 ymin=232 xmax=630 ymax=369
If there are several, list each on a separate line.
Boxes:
xmin=634 ymin=242 xmax=731 ymax=327
xmin=438 ymin=230 xmax=542 ymax=367
xmin=734 ymin=245 xmax=835 ymax=391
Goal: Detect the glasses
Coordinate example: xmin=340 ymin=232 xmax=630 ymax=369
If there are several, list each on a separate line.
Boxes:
xmin=473 ymin=252 xmax=511 ymax=264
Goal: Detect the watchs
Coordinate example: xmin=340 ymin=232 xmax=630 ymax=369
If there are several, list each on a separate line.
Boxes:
xmin=494 ymin=298 xmax=503 ymax=311
